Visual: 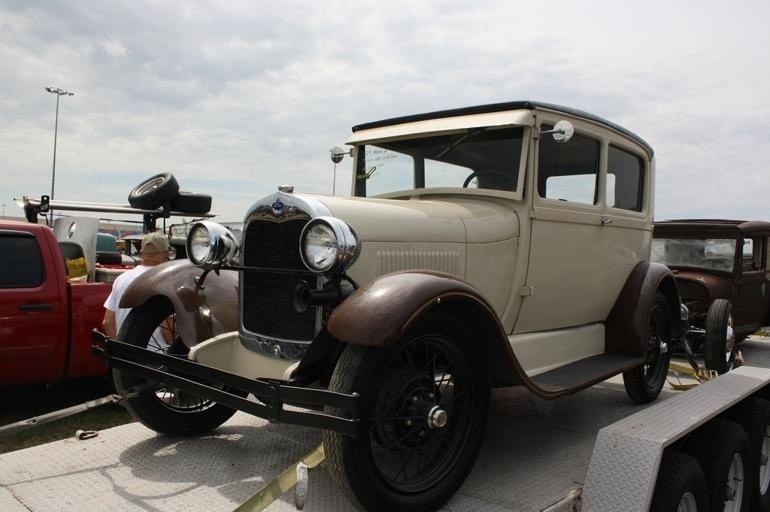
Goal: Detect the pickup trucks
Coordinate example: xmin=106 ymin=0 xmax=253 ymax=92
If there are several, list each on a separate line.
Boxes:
xmin=1 ymin=218 xmax=113 ymax=391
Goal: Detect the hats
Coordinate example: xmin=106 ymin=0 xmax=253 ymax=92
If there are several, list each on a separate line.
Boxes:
xmin=140 ymin=232 xmax=170 ymax=254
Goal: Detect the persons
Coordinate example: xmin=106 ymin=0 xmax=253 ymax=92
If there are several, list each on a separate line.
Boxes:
xmin=102 ymin=232 xmax=178 ymax=354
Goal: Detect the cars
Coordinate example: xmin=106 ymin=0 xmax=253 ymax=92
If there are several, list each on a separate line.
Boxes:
xmin=647 ymin=219 xmax=769 ymax=374
xmin=90 ymin=100 xmax=683 ymax=512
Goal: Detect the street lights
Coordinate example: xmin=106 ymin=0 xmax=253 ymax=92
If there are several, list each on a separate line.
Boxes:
xmin=42 ymin=84 xmax=76 ymax=226
